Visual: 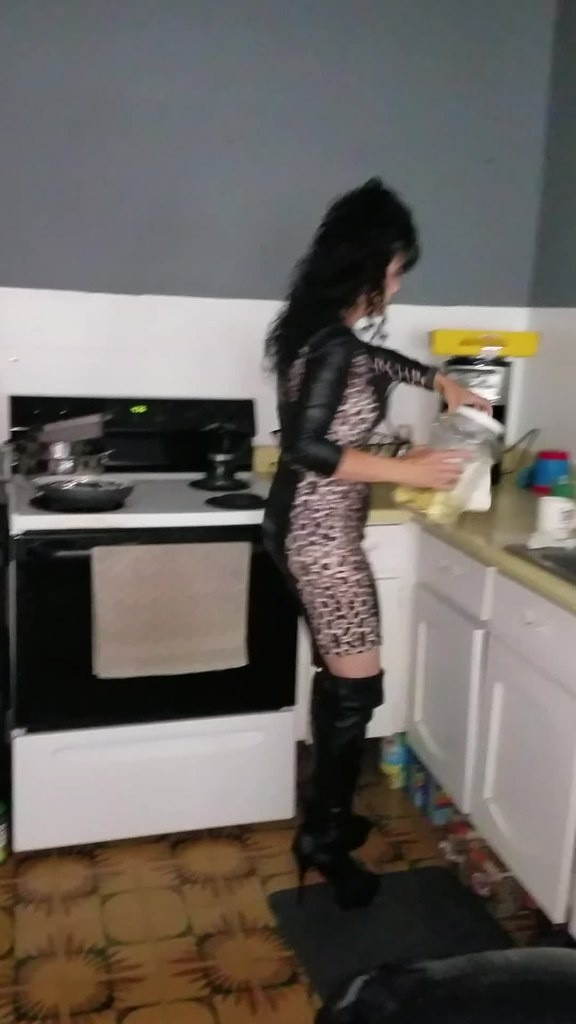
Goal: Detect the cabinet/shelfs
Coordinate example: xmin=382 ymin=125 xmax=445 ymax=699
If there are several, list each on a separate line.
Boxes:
xmin=296 ymin=520 xmax=576 ymax=924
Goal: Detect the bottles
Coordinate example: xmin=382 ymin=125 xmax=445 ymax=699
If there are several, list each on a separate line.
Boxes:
xmin=553 ymin=475 xmax=573 ymax=499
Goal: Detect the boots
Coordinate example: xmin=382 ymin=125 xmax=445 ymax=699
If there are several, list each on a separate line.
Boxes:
xmin=349 ymin=809 xmax=377 ymax=846
xmin=290 ymin=667 xmax=388 ymax=909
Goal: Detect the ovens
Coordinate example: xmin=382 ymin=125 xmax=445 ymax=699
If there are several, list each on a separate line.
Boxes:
xmin=15 ymin=526 xmax=301 ymax=726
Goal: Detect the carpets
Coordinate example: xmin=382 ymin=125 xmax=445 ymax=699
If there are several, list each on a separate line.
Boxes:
xmin=265 ymin=865 xmax=518 ymax=1004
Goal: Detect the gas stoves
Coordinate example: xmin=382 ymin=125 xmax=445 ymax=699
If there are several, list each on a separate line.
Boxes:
xmin=9 ymin=396 xmax=275 ymax=526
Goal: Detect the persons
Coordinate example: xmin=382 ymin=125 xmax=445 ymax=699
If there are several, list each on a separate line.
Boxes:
xmin=262 ymin=175 xmax=493 ymax=908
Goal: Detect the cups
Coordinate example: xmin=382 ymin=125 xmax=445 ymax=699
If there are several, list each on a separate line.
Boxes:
xmin=531 ymin=451 xmax=570 ymax=495
xmin=533 ymin=496 xmax=576 ymax=541
xmin=206 ymin=453 xmax=235 ymax=483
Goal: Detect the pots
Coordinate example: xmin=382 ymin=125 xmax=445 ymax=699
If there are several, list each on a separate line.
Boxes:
xmin=40 ymin=475 xmax=134 ymax=508
xmin=33 ymin=441 xmax=121 ymax=478
